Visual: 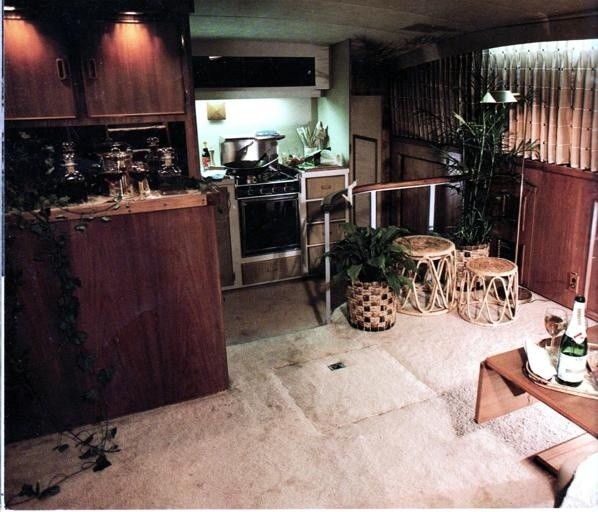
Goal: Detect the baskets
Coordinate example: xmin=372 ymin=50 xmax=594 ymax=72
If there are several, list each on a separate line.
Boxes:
xmin=343 ymin=276 xmax=396 ymax=331
xmin=445 ymin=244 xmax=491 ymax=291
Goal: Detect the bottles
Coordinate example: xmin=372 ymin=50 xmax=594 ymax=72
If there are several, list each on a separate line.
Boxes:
xmin=555 ymin=297 xmax=588 ymax=386
xmin=201 ymin=142 xmax=215 ymax=170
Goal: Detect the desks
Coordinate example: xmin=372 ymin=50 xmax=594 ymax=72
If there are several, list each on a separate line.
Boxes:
xmin=475 ymin=321 xmax=598 ymax=470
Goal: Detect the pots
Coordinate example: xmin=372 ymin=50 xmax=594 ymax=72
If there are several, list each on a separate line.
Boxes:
xmin=220 ymin=131 xmax=285 ymax=163
xmin=207 ymin=161 xmax=268 ymax=176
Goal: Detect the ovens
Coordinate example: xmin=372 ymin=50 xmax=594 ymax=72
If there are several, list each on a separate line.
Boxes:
xmin=238 ymin=194 xmax=302 ymax=285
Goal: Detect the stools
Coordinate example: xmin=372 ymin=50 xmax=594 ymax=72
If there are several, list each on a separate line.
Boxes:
xmin=459 ymin=259 xmax=518 ymax=326
xmin=393 ymin=233 xmax=458 ymax=316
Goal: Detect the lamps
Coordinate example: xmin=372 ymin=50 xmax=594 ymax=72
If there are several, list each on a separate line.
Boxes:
xmin=479 ymin=88 xmax=523 ymax=104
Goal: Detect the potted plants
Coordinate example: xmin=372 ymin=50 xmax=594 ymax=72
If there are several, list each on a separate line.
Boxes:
xmin=427 ymin=68 xmax=531 ymax=259
xmin=315 ymin=225 xmax=415 ymax=332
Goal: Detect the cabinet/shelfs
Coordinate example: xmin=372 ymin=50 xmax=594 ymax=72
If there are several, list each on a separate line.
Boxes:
xmin=199 ymin=177 xmax=355 ymax=291
xmin=388 ymin=141 xmax=598 ymax=319
xmin=68 ymin=0 xmax=194 ymax=126
xmin=2 ymin=1 xmax=78 ymax=125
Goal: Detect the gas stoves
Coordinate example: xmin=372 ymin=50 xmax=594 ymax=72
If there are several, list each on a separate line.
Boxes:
xmin=222 ymin=165 xmax=302 ymax=198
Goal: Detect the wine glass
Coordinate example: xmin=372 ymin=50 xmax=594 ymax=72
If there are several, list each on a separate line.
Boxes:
xmin=544 ymin=307 xmax=568 ymax=355
